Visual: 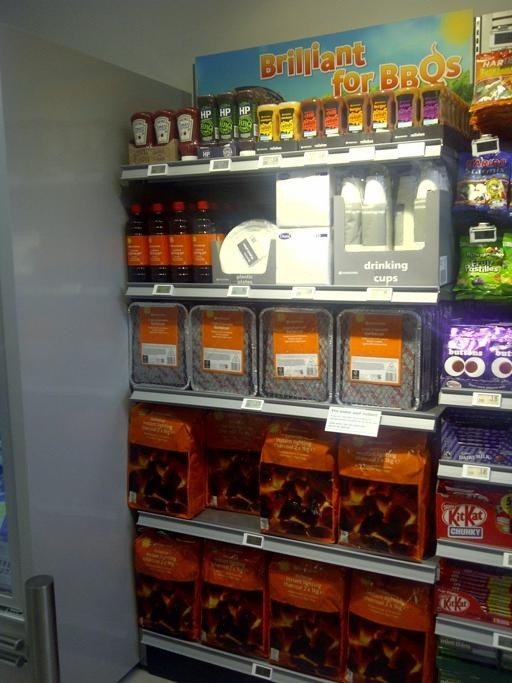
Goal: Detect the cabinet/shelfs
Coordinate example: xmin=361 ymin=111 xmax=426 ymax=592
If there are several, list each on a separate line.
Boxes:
xmin=120 ymin=137 xmax=463 ymax=682
xmin=421 ymin=386 xmax=512 ymax=656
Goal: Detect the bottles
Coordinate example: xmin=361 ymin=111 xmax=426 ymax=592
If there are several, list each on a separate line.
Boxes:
xmin=126 ymin=201 xmax=218 ymax=285
xmin=131 ymin=87 xmax=470 ymax=165
